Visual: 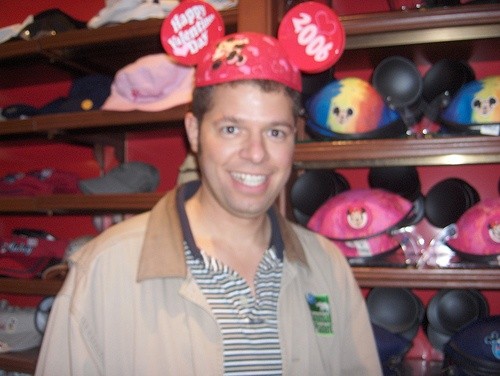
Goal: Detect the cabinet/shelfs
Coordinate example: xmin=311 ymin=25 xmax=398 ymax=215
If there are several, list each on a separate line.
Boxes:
xmin=0 ymin=0 xmax=500 ymax=376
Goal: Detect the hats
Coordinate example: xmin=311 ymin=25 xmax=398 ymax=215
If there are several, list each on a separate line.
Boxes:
xmin=0 ymin=0 xmax=198 ymax=354
xmin=290 ymin=39 xmax=500 ymax=376
xmin=161 ymin=0 xmax=346 ymax=93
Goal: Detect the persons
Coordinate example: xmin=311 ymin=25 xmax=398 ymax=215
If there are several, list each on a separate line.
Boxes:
xmin=34 ymin=0 xmax=381 ymax=375
